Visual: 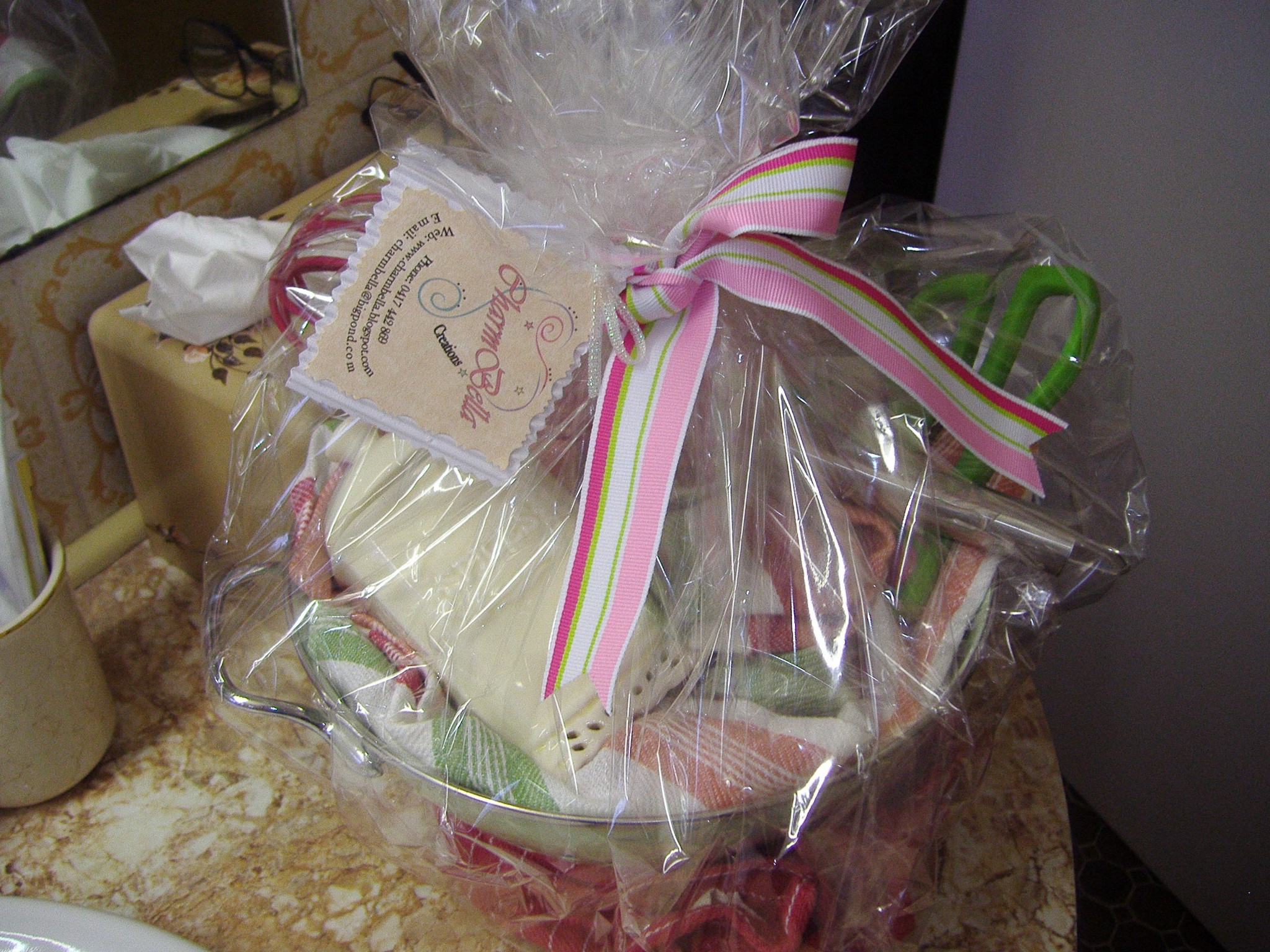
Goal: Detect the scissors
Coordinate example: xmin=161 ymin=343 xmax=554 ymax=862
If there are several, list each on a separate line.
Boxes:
xmin=878 ymin=267 xmax=1100 ymax=622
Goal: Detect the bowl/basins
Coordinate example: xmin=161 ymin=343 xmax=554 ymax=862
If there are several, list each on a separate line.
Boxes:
xmin=199 ymin=471 xmax=1108 ymax=871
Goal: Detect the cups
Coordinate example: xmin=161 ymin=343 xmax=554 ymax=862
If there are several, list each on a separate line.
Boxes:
xmin=0 ymin=517 xmax=120 ymax=807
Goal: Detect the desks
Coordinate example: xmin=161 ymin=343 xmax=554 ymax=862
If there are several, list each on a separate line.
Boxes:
xmin=0 ymin=530 xmax=1081 ymax=952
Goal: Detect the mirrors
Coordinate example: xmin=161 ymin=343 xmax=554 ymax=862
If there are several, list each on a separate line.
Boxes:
xmin=0 ymin=0 xmax=311 ymax=262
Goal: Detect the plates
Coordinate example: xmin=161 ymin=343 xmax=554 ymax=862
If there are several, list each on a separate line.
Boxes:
xmin=0 ymin=895 xmax=207 ymax=952
